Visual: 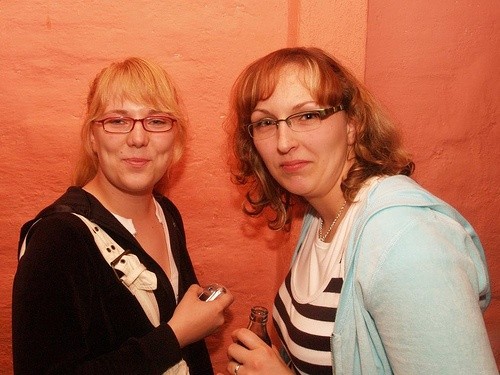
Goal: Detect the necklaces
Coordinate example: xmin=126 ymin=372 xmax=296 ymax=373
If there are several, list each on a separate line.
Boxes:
xmin=319 ymin=198 xmax=347 ymax=242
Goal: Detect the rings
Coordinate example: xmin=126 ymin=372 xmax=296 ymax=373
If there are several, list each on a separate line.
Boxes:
xmin=234 ymin=365 xmax=240 ymax=375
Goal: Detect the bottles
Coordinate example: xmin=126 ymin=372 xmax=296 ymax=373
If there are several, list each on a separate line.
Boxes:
xmin=239 ymin=306 xmax=272 ymax=350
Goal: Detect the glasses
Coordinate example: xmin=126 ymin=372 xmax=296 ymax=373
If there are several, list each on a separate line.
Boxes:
xmin=246 ymin=105 xmax=345 ymax=138
xmin=90 ymin=116 xmax=180 ymax=133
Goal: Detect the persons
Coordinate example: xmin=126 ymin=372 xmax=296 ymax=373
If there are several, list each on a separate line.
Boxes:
xmin=226 ymin=48 xmax=500 ymax=375
xmin=10 ymin=57 xmax=234 ymax=375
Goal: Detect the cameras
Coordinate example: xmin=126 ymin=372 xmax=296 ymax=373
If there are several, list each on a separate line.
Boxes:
xmin=198 ymin=284 xmax=227 ymax=302
